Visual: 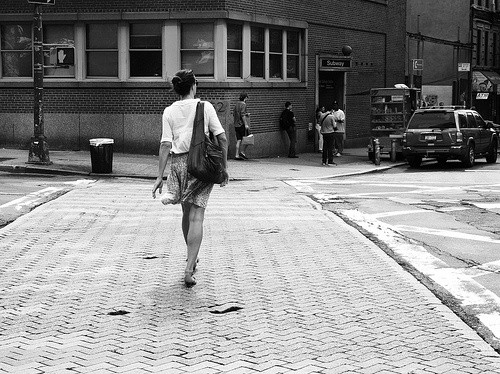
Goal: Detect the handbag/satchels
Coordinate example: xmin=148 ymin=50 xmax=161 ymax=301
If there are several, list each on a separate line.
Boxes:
xmin=187 ymin=102 xmax=224 ymax=183
xmin=242 ymin=126 xmax=255 ymax=145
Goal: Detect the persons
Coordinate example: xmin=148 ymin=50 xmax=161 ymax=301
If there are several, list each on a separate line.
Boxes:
xmin=233 ymin=92 xmax=251 ymax=160
xmin=332 ymin=101 xmax=345 ymax=157
xmin=320 ymin=104 xmax=337 ymax=165
xmin=152 ymin=68 xmax=229 ymax=286
xmin=316 ymin=105 xmax=325 ymax=152
xmin=282 ymin=101 xmax=299 ymax=158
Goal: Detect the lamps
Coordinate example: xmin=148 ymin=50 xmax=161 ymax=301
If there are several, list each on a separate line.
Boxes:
xmin=343 ymin=45 xmax=353 ymax=56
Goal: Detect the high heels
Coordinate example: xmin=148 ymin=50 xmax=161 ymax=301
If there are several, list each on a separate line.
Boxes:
xmin=185 ymin=258 xmax=199 ymax=270
xmin=184 ymin=271 xmax=196 ymax=287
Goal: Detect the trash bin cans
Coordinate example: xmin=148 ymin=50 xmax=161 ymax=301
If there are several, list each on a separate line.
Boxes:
xmin=89 ymin=139 xmax=113 ymax=173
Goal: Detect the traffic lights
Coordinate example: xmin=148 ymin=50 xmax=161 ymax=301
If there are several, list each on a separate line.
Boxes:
xmin=56 ymin=47 xmax=75 ymax=65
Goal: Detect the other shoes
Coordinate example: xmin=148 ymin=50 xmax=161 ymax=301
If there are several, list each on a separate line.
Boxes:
xmin=288 ymin=155 xmax=293 ymax=158
xmin=322 ymin=162 xmax=326 ymax=166
xmin=235 ymin=157 xmax=244 ymax=160
xmin=239 ymin=152 xmax=248 ymax=159
xmin=336 ymin=153 xmax=342 ymax=157
xmin=328 ymin=163 xmax=337 ymax=166
xmin=290 ymin=156 xmax=299 ymax=158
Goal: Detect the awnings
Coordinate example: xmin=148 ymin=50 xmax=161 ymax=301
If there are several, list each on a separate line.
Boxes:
xmin=473 ymin=70 xmax=500 ymax=93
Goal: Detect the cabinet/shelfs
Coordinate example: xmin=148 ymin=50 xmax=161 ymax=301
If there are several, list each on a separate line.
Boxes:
xmin=370 ymin=88 xmax=410 ymax=153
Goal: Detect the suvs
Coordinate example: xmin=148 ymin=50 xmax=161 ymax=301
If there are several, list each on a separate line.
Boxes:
xmin=403 ymin=106 xmax=498 ymax=168
